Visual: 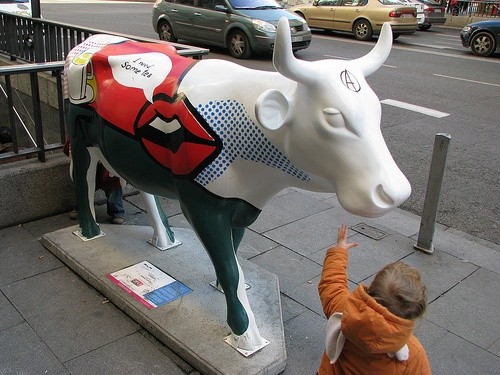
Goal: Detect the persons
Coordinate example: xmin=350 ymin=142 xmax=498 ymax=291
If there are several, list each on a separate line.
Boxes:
xmin=438 ymin=0 xmax=498 ymax=17
xmin=317 ymin=222 xmax=431 ymax=375
xmin=62 ymin=128 xmax=124 ymax=225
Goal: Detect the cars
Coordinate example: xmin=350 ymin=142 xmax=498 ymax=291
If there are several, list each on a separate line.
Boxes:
xmin=151 ymin=0 xmax=313 ymax=60
xmin=289 ymin=0 xmax=419 ymax=41
xmin=460 ymin=20 xmax=500 ymax=57
xmin=404 ymin=0 xmax=446 ymax=30
xmin=0 ymin=0 xmax=46 ymax=48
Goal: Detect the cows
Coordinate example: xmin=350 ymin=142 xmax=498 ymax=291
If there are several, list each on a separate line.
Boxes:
xmin=62 ymin=17 xmax=413 ymax=359
